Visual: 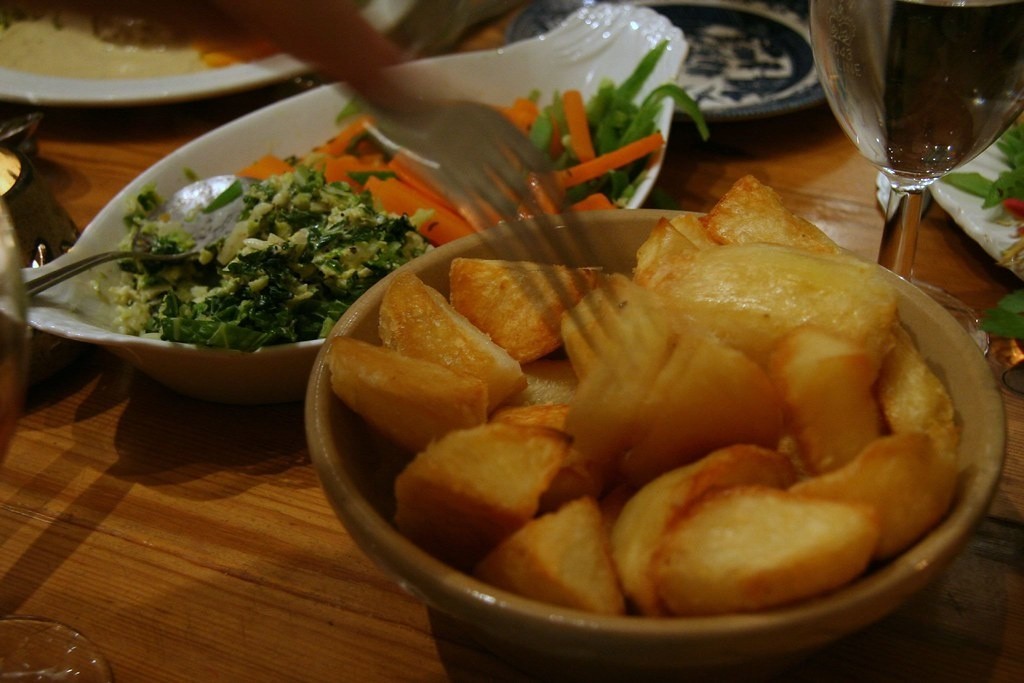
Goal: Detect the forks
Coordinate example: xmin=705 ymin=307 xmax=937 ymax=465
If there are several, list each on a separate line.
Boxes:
xmin=215 ymin=1 xmax=622 ymax=356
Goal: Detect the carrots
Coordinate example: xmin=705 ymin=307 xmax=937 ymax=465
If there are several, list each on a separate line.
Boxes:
xmin=240 ymin=89 xmax=664 ymax=249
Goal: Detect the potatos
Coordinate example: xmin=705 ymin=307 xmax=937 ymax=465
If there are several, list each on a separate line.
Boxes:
xmin=325 ymin=172 xmax=958 ymax=617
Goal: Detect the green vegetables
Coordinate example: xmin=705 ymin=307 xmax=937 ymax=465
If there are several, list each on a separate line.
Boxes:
xmin=527 ymin=39 xmax=709 ymax=206
xmin=938 ymin=113 xmax=1024 ymax=344
xmin=113 ymin=156 xmax=439 ymax=353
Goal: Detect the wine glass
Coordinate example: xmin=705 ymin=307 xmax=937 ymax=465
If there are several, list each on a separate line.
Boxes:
xmin=1 ymin=209 xmax=111 ymax=683
xmin=807 ymin=1 xmax=1024 ymax=360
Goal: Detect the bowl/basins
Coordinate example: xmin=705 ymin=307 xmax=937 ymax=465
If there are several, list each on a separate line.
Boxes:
xmin=303 ymin=209 xmax=1006 ymax=671
xmin=14 ymin=5 xmax=689 ymax=403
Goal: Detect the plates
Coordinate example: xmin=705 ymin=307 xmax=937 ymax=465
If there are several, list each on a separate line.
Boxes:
xmin=928 ymin=134 xmax=1024 ymax=282
xmin=505 ymin=1 xmax=833 ymax=121
xmin=1 ymin=0 xmax=408 ymax=110
